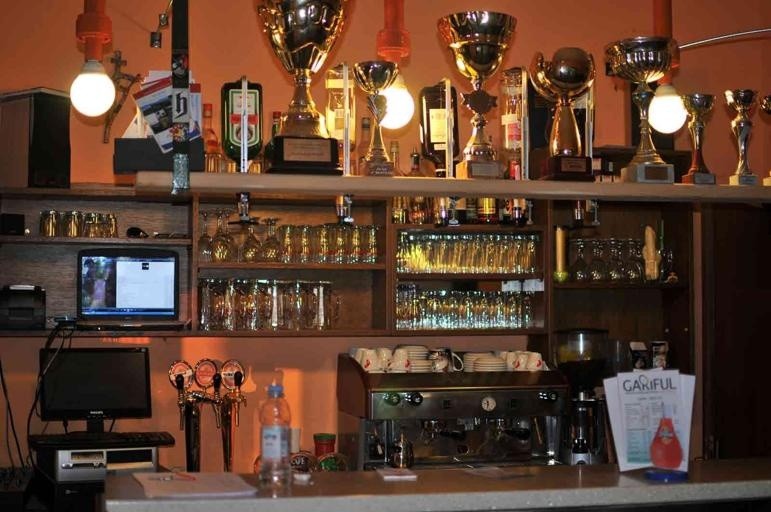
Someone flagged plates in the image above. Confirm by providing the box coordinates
[465,349,506,371]
[403,344,431,373]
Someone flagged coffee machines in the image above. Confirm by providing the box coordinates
[554,326,609,466]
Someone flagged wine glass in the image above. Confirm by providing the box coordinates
[569,237,678,282]
[201,206,282,262]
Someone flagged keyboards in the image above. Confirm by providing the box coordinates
[30,432,175,447]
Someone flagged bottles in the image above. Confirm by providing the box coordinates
[41,210,116,240]
[388,139,405,177]
[420,85,462,177]
[281,223,378,263]
[326,61,372,174]
[396,230,540,273]
[190,75,282,173]
[259,384,295,493]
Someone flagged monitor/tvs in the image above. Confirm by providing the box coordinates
[38,346,152,435]
[76,247,185,332]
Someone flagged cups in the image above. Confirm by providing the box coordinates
[428,349,467,371]
[350,345,410,374]
[496,349,545,371]
[200,276,343,331]
[395,283,533,329]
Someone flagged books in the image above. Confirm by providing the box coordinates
[376,466,416,481]
[601,366,696,474]
[132,471,259,498]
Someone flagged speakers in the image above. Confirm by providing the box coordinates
[0,87,71,189]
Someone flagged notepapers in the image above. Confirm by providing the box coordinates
[376,468,419,483]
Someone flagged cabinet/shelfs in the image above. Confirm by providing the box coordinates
[0,183,709,466]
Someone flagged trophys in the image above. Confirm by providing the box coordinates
[255,2,345,176]
[437,11,517,179]
[757,95,771,186]
[725,88,759,185]
[680,92,717,185]
[603,36,680,186]
[352,60,399,177]
[418,77,459,178]
[322,62,359,173]
[529,46,599,184]
